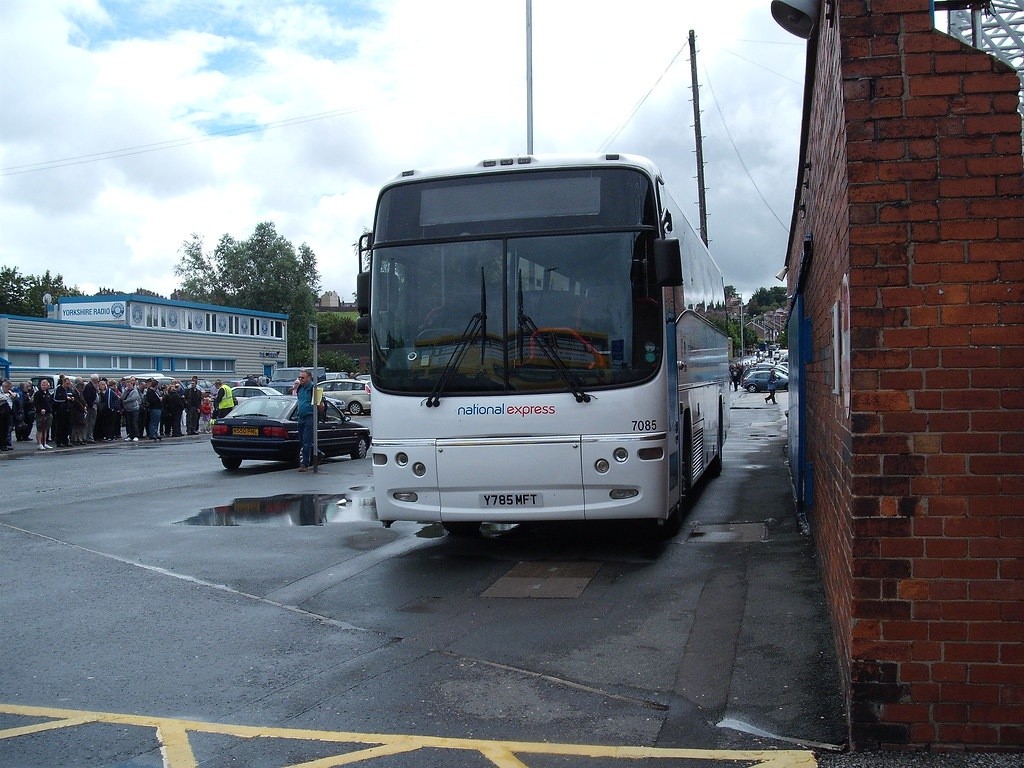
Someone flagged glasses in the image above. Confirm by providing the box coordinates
[299,377,308,379]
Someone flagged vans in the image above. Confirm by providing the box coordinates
[31,373,78,401]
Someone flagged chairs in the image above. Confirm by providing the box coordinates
[459,286,613,324]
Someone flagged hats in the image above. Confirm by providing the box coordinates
[212,379,222,386]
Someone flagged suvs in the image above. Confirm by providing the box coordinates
[122,373,212,398]
[739,361,789,393]
[316,378,371,416]
[266,366,326,394]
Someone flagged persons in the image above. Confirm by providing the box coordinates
[728,362,745,391]
[297,371,326,472]
[764,370,778,404]
[0,372,356,452]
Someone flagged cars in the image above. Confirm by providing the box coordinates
[210,395,371,470]
[326,372,371,390]
[231,385,284,405]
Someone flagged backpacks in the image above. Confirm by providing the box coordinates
[296,384,328,424]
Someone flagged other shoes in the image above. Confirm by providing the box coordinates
[73,441,87,446]
[44,444,53,448]
[23,437,33,441]
[298,464,306,472]
[37,444,46,449]
[0,446,13,451]
[17,438,23,442]
[188,431,199,435]
[57,443,73,447]
[150,435,164,439]
[103,438,118,442]
[204,431,211,433]
[124,437,132,441]
[133,437,139,441]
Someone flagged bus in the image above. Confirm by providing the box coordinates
[356,153,730,539]
